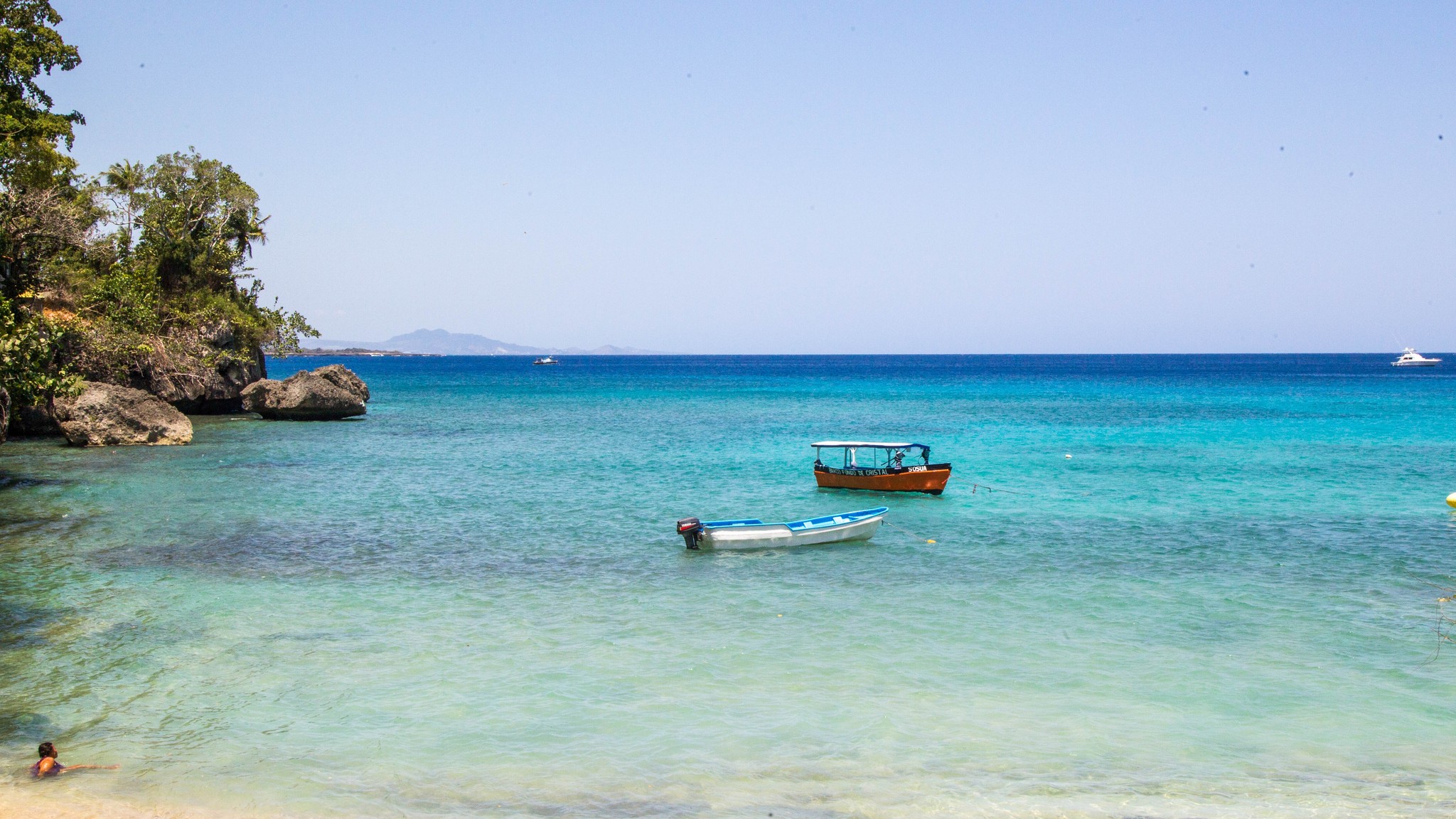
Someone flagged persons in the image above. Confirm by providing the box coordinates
[33,743,120,776]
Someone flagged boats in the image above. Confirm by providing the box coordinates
[532,354,558,366]
[1390,349,1443,367]
[674,503,891,556]
[808,440,953,494]
